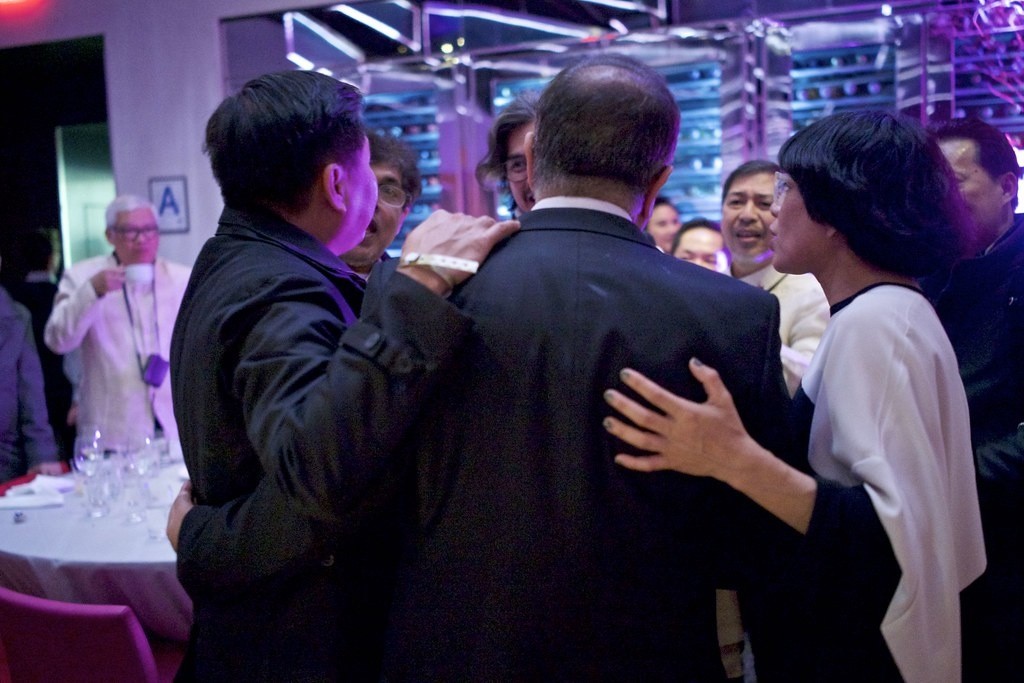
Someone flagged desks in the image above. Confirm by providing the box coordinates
[0,446,198,642]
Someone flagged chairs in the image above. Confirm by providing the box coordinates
[0,585,185,683]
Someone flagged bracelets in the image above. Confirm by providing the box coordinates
[397,252,480,299]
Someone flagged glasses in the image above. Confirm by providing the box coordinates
[772,171,803,205]
[378,184,412,208]
[112,225,159,242]
[502,155,527,182]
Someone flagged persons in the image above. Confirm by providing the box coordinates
[0,70,1024,682]
[165,49,790,683]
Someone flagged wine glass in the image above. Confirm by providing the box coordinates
[73,423,191,540]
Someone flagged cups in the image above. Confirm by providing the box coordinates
[119,263,153,286]
